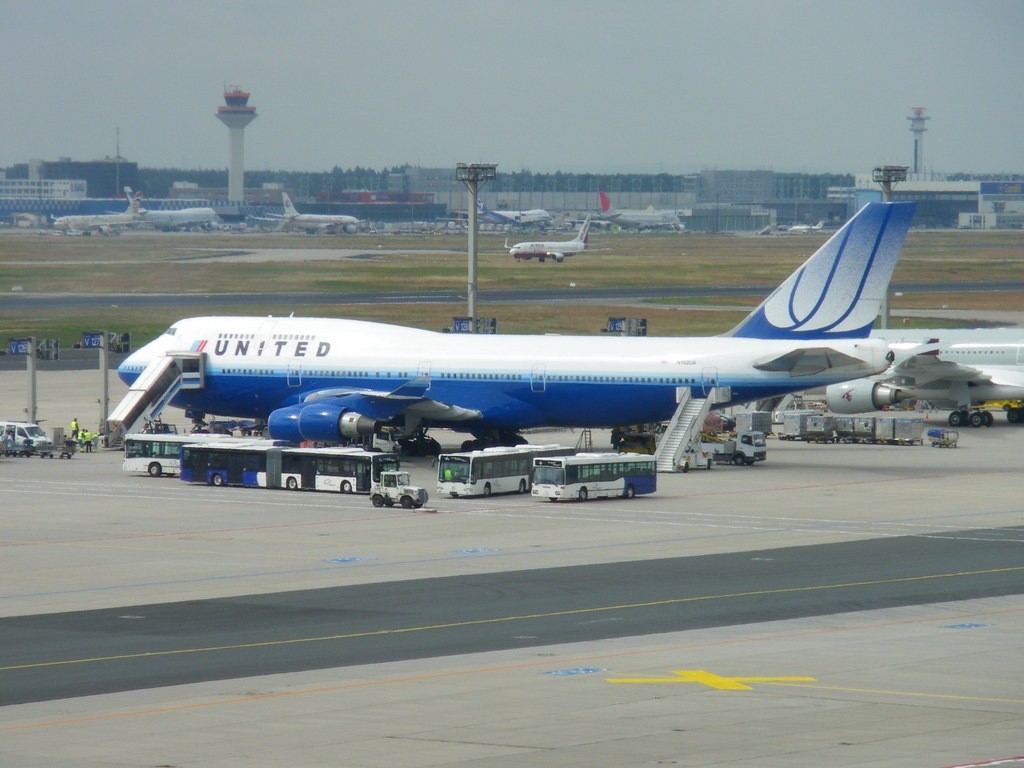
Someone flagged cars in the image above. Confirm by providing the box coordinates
[369,469,428,509]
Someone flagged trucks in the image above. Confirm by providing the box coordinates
[927,429,959,448]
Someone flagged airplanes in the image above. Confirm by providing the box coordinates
[771,327,1024,427]
[504,213,594,264]
[281,191,361,235]
[123,184,222,232]
[598,191,687,232]
[475,198,557,232]
[788,220,825,234]
[52,190,142,237]
[110,198,920,472]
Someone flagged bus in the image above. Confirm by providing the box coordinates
[431,444,575,500]
[122,433,291,477]
[180,442,401,495]
[531,452,657,501]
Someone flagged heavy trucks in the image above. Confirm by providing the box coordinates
[657,429,767,465]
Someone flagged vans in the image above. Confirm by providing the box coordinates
[0,421,47,448]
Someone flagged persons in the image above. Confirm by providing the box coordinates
[77,428,86,453]
[92,432,99,453]
[6,432,15,448]
[70,417,79,440]
[84,429,92,453]
[443,467,452,481]
